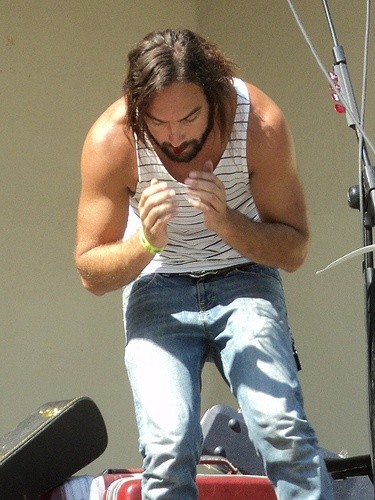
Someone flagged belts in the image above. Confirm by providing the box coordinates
[162,264,246,277]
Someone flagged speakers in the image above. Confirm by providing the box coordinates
[198,404,341,477]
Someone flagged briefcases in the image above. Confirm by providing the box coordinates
[0,396,375,499]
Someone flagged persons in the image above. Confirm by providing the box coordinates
[73,29,338,500]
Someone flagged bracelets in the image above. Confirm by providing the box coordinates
[138,227,167,254]
